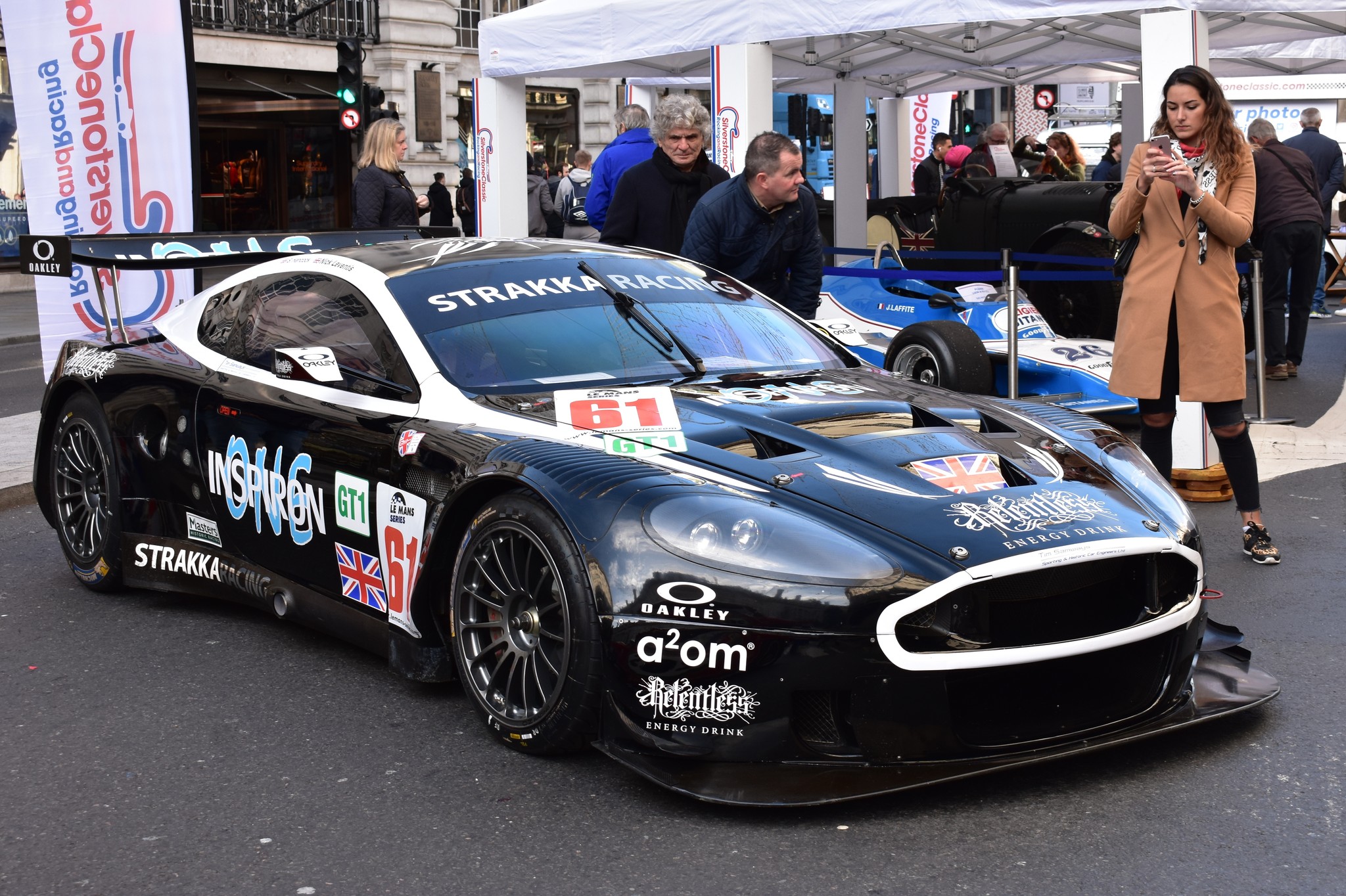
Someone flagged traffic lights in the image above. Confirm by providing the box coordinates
[367,84,401,130]
[334,37,363,133]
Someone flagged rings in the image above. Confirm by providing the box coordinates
[1153,165,1156,172]
[1184,161,1188,168]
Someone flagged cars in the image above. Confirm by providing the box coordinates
[800,163,1265,358]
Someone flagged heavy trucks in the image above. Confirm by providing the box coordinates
[772,91,878,198]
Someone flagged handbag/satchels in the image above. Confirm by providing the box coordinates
[1114,217,1142,277]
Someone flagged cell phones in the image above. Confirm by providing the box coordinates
[1031,143,1048,152]
[1150,135,1172,175]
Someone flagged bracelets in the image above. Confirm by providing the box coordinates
[1190,187,1205,205]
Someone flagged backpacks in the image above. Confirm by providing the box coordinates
[456,182,476,216]
[561,175,592,226]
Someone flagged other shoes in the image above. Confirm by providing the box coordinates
[1286,360,1297,377]
[1254,363,1288,380]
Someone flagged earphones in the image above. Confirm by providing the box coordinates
[939,147,941,151]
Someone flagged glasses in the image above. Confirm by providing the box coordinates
[1053,132,1070,146]
[988,136,1007,144]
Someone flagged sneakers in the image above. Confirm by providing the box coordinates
[1242,521,1280,564]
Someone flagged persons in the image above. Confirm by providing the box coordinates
[598,91,730,256]
[351,116,434,230]
[680,132,823,320]
[553,149,602,242]
[526,150,570,239]
[1012,130,1086,182]
[1247,117,1324,381]
[914,132,953,197]
[942,103,1022,210]
[584,102,659,235]
[1107,64,1282,565]
[1091,131,1121,182]
[1282,107,1346,318]
[455,167,476,237]
[427,172,455,227]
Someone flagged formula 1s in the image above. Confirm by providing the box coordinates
[810,240,1173,418]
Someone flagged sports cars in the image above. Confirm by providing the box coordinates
[20,224,1282,809]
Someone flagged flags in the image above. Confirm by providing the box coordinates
[0,0,197,385]
